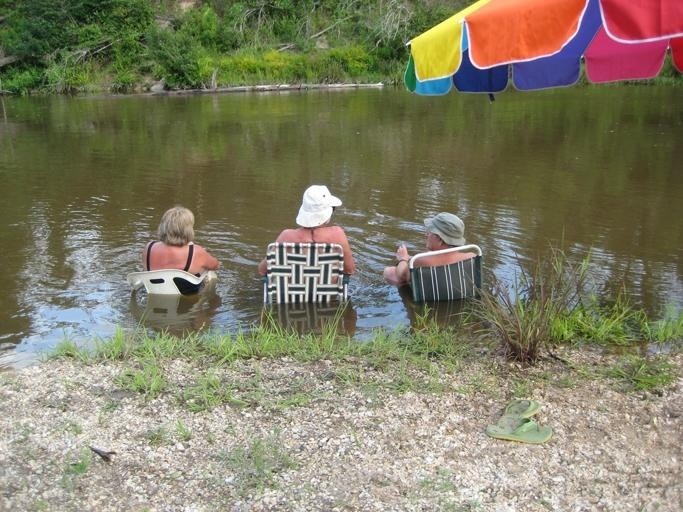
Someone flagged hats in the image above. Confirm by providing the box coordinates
[424,211,466,246]
[296,185,342,228]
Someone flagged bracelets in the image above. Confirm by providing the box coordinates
[398,260,407,263]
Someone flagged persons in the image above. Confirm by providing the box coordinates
[382,211,476,288]
[257,183,356,286]
[141,204,219,296]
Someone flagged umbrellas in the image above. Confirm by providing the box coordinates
[402,1,683,104]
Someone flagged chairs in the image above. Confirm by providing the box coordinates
[262,242,349,302]
[127,268,217,296]
[408,244,484,302]
